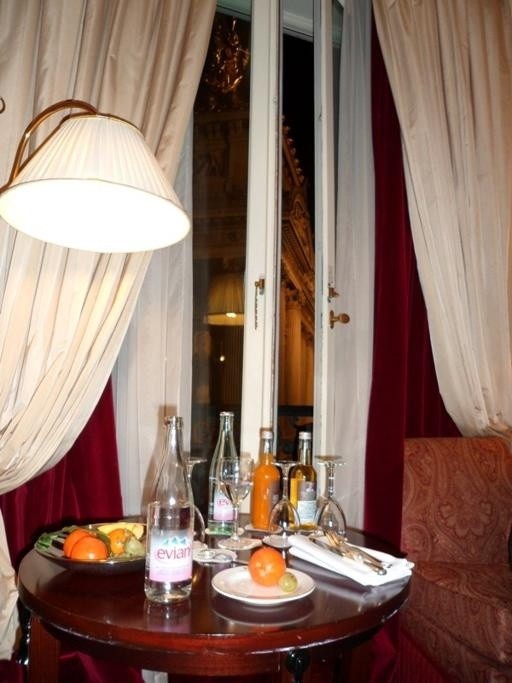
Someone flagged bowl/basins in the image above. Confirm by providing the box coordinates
[35,520,145,576]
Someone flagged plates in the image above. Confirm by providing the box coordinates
[210,565,315,605]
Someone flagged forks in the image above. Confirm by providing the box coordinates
[326,531,383,564]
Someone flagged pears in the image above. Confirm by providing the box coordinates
[123,525,146,558]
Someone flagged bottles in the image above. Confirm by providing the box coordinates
[206,412,319,537]
[147,414,195,604]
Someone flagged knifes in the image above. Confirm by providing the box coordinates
[306,538,385,578]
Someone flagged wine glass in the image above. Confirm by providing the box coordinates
[318,458,348,539]
[182,456,210,547]
[267,459,301,545]
[220,457,255,550]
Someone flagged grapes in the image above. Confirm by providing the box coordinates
[279,574,297,592]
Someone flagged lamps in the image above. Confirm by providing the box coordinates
[0,97,192,255]
[202,272,244,326]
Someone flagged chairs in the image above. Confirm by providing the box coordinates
[399,434,511,683]
